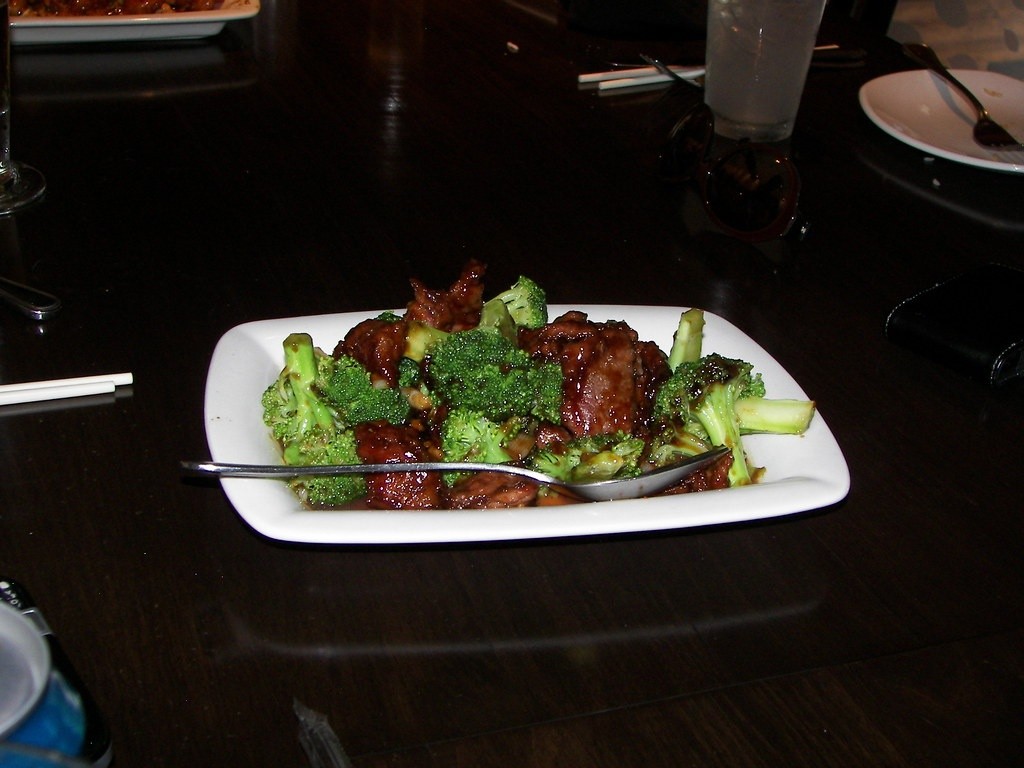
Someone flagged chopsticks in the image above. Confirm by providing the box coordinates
[578,65,707,90]
[0,372,133,406]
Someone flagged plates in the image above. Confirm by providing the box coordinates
[12,0,261,47]
[204,303,850,543]
[858,68,1024,173]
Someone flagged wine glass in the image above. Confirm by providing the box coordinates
[0,0,47,217]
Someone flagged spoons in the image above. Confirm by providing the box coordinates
[180,445,735,499]
[640,49,865,89]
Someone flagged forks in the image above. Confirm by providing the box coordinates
[903,39,1024,149]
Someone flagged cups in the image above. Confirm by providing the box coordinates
[704,0,826,143]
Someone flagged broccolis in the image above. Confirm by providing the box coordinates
[260,274,816,505]
